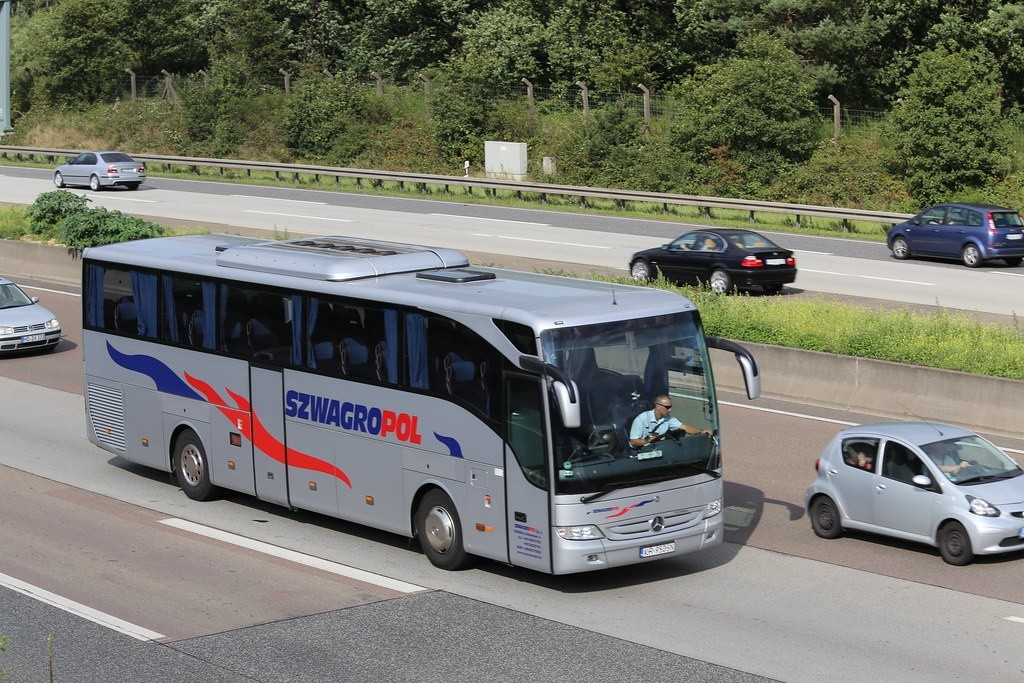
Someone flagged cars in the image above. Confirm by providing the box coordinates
[52,150,147,191]
[886,202,1024,268]
[629,228,797,296]
[805,420,1024,567]
[0,278,63,356]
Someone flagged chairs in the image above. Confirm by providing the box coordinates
[104,295,489,399]
[705,239,717,249]
[606,374,653,453]
[886,444,915,482]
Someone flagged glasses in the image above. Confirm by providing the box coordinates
[657,403,672,410]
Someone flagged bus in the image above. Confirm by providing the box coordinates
[81,233,762,577]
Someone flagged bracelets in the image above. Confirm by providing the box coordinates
[700,429,703,434]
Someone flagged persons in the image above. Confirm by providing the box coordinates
[680,235,708,250]
[921,441,971,489]
[854,451,871,469]
[628,395,713,449]
